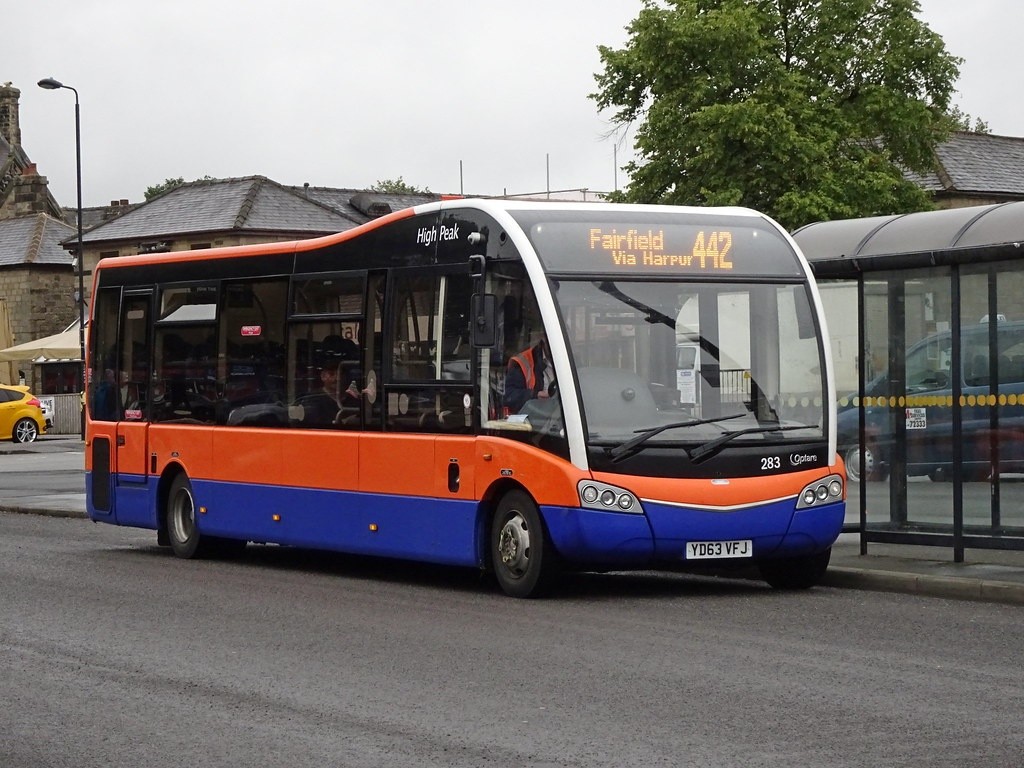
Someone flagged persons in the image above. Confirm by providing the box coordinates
[503,319,570,415]
[96,333,358,430]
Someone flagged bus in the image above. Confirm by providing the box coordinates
[85,196,848,600]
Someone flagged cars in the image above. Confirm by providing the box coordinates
[0,382,48,444]
[836,313,1023,485]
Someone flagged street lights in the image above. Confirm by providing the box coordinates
[36,76,85,442]
